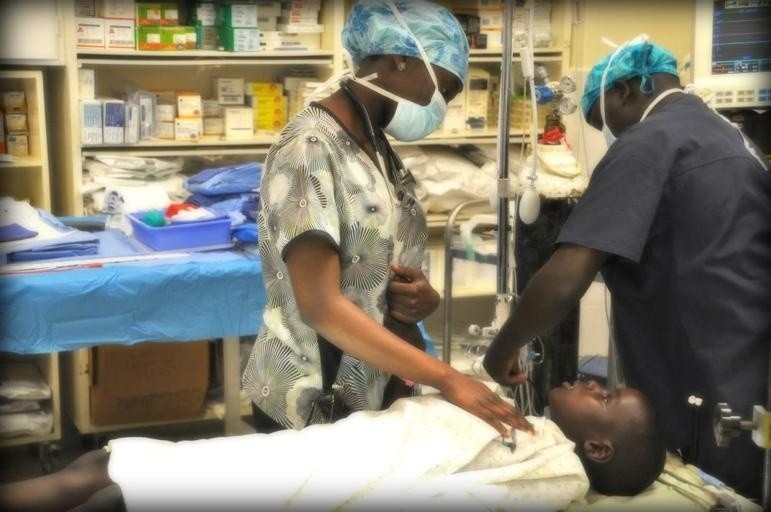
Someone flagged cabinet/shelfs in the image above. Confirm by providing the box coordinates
[74,1,344,434]
[72,60,341,147]
[349,3,568,304]
[67,0,343,60]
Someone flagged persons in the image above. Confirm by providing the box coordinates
[239,1,537,443]
[4,372,670,510]
[482,36,771,507]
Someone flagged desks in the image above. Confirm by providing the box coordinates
[3,249,274,357]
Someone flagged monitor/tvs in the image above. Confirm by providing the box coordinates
[693,0,771,110]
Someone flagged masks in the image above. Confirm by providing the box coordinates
[355,27,448,143]
[599,86,653,146]
